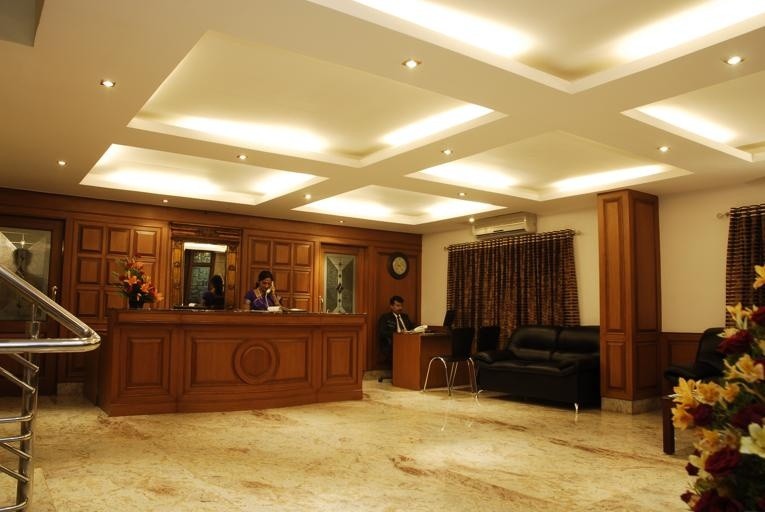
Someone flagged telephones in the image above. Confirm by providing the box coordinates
[414,325,428,333]
[266,281,274,294]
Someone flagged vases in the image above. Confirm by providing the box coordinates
[127,293,144,308]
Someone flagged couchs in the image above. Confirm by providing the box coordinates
[478,325,599,412]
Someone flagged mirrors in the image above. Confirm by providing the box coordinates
[164,222,243,310]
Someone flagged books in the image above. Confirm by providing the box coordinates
[403,323,427,334]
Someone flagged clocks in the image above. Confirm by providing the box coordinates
[388,253,410,279]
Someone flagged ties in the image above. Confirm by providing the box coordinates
[396,316,404,332]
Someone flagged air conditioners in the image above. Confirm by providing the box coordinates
[472,212,537,241]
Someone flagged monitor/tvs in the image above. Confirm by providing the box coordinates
[443,309,457,326]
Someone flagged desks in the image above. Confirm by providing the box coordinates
[392,331,476,390]
[660,395,682,455]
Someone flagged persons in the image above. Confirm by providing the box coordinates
[201,273,225,311]
[1,246,44,317]
[243,268,283,312]
[375,294,415,371]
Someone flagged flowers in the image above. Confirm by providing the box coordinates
[115,255,164,303]
[669,265,765,512]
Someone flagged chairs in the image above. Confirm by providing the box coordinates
[661,328,765,393]
[452,325,499,393]
[423,327,475,396]
[377,336,393,382]
